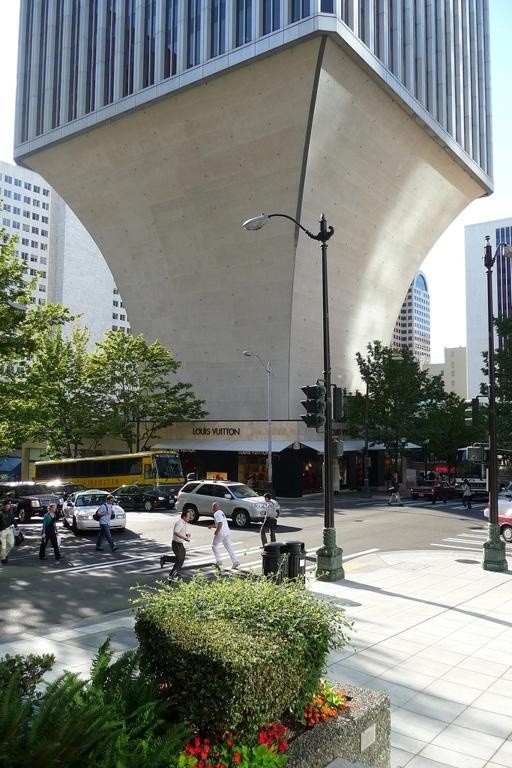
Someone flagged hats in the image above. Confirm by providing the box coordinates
[3,500,12,505]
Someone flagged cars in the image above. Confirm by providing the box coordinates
[497,507,512,542]
[61,488,126,533]
[109,481,175,510]
[410,479,454,500]
[484,498,512,521]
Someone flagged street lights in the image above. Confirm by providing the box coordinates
[480,234,511,526]
[243,206,350,583]
[241,348,276,491]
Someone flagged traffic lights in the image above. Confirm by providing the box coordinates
[462,398,475,428]
[299,381,327,430]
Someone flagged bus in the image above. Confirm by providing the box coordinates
[452,443,512,496]
[32,449,187,494]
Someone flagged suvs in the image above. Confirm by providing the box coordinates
[37,483,88,505]
[0,481,64,522]
[173,478,282,526]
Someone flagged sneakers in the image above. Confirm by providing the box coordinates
[160,554,166,569]
[232,560,240,570]
[95,545,120,552]
[39,556,64,560]
[215,560,224,567]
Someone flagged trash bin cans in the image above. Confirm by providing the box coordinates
[261,541,291,588]
[286,540,308,584]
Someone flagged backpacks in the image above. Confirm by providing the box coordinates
[92,502,109,521]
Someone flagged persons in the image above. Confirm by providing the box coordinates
[161,512,190,578]
[461,479,471,510]
[260,494,277,549]
[0,503,18,563]
[247,476,256,488]
[40,504,64,560]
[96,495,119,551]
[208,502,240,569]
[418,471,436,485]
[388,473,404,506]
